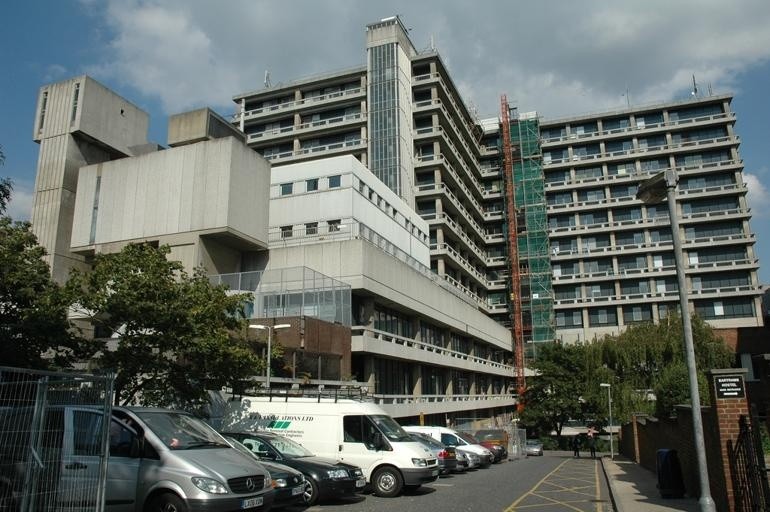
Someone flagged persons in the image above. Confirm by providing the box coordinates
[589,440,598,459]
[573,437,582,459]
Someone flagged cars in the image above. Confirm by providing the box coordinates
[401,425,510,476]
[220,431,367,512]
[522,439,544,457]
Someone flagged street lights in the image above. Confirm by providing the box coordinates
[600,383,614,461]
[634,169,717,512]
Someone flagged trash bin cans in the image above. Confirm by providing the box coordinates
[656,449,687,500]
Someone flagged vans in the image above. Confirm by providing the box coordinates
[202,393,440,498]
[0,405,275,512]
